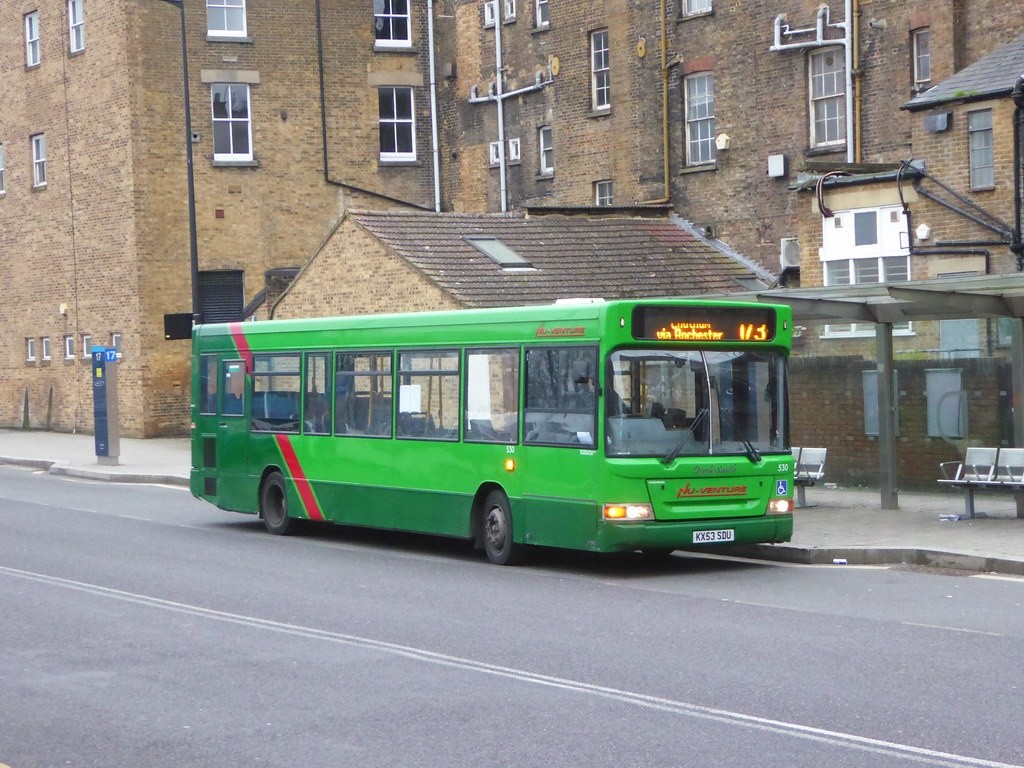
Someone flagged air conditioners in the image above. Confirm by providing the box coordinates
[781,237,800,271]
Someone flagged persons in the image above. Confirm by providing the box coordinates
[575,372,629,419]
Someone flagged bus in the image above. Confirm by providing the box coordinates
[186,300,795,565]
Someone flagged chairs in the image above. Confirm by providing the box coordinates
[794,447,827,480]
[1002,464,1024,486]
[264,390,291,419]
[937,447,998,483]
[352,391,380,429]
[791,447,801,468]
[240,392,264,419]
[377,391,392,422]
[208,392,238,414]
[968,447,1024,485]
[409,411,436,437]
[470,419,496,440]
